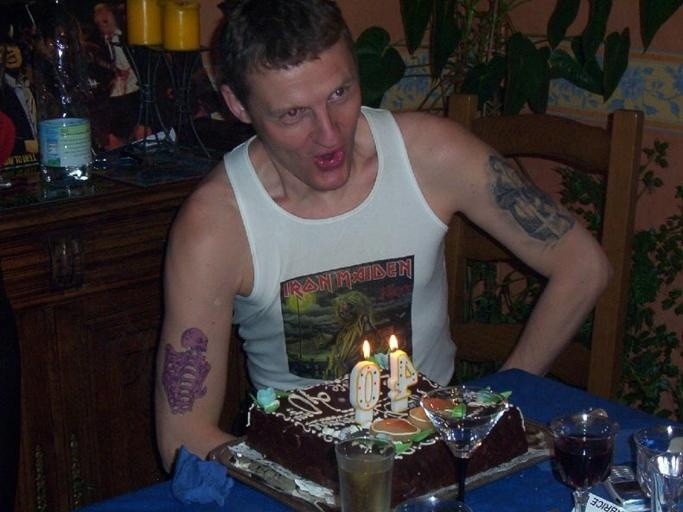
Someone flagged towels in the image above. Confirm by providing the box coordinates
[172,447,234,507]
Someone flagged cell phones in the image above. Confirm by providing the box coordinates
[603,464,646,508]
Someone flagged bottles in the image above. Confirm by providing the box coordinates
[31,1,94,188]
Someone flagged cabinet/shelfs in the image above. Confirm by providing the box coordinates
[1,159,257,511]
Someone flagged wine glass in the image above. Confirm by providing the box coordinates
[420,387,508,510]
[550,413,621,511]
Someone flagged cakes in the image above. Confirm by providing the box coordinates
[244,363,529,512]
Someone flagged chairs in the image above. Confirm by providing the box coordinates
[444,94,646,399]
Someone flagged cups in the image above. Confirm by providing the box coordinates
[648,452,682,512]
[632,425,683,499]
[335,438,395,512]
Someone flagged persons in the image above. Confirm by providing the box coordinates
[88,2,157,152]
[0,35,49,152]
[153,1,619,479]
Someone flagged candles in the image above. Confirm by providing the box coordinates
[125,0,201,51]
[386,334,419,413]
[349,340,381,428]
